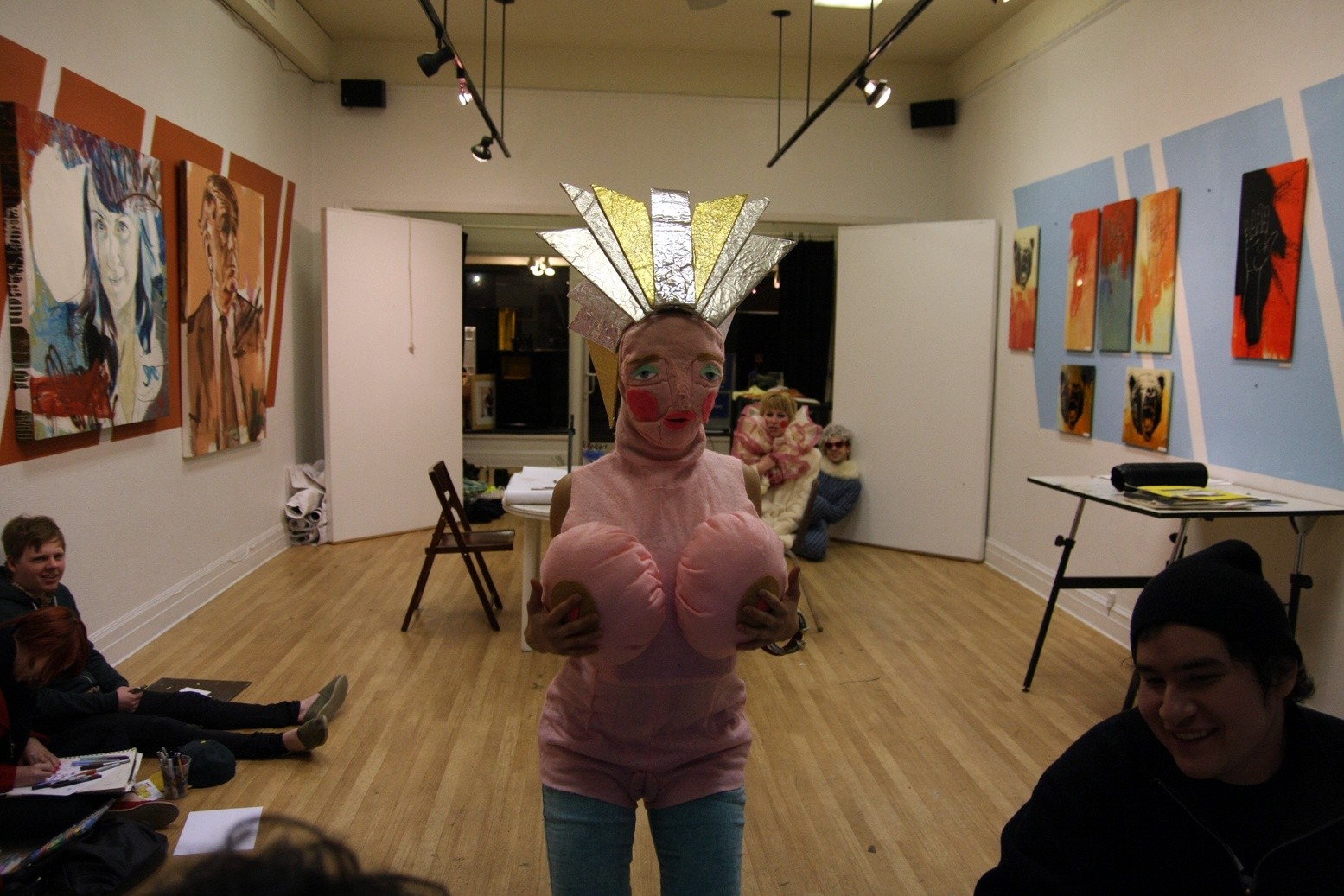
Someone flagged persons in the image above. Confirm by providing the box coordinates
[523,308,862,896]
[975,539,1344,895]
[0,517,447,896]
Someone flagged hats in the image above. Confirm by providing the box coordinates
[1130,538,1302,664]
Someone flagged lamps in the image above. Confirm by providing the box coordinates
[767,0,933,167]
[528,254,555,276]
[416,0,514,163]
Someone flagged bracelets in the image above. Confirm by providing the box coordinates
[764,611,807,656]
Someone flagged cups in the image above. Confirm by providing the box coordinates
[160,755,191,799]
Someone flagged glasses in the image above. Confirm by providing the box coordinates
[824,441,844,448]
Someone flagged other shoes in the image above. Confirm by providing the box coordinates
[304,674,349,722]
[292,715,328,758]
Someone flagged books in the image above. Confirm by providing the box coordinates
[6,747,143,798]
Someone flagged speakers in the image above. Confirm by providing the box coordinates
[340,78,387,108]
[909,98,957,129]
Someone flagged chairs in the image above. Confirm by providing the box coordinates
[399,461,516,632]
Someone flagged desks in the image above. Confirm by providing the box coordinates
[1020,476,1344,693]
[502,466,590,652]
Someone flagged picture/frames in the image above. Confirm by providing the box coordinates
[471,376,495,431]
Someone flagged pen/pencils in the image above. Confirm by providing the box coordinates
[130,684,148,692]
[31,763,121,789]
[156,746,187,798]
[30,753,38,763]
[71,755,130,770]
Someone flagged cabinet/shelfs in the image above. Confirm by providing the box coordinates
[496,349,568,435]
